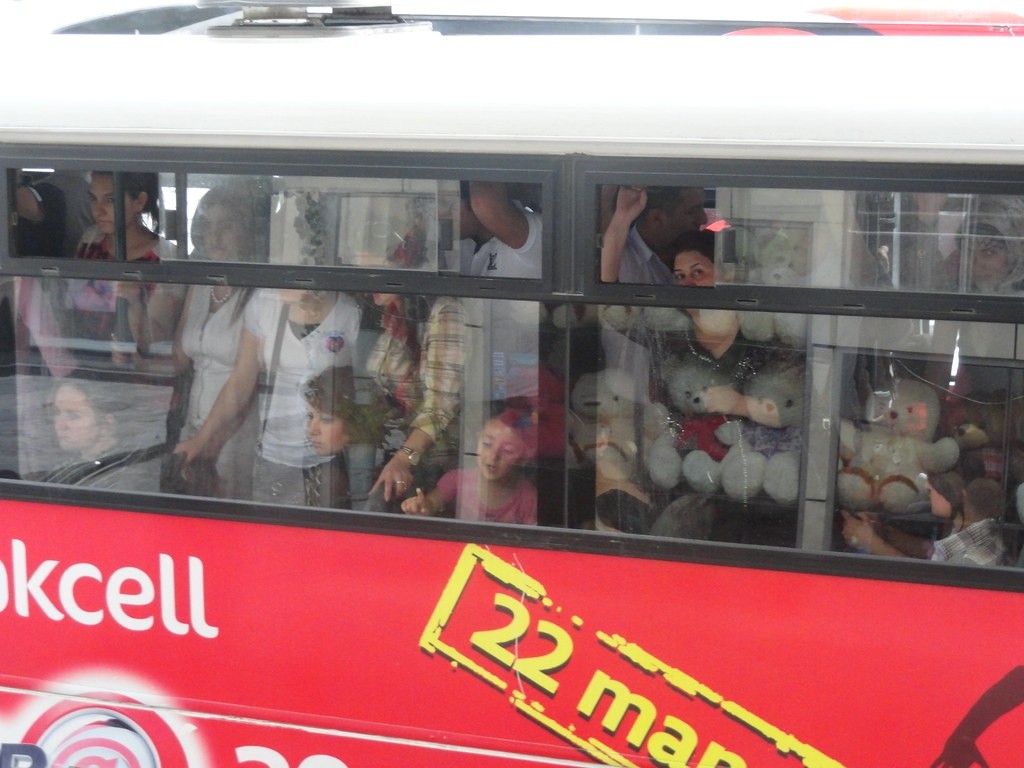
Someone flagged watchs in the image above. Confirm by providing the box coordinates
[400,446,419,465]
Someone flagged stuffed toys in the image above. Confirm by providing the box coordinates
[648,363,737,489]
[719,360,799,501]
[569,372,659,480]
[836,378,960,513]
[941,390,1024,517]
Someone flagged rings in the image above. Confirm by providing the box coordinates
[397,481,404,484]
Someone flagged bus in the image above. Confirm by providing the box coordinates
[0,0,1024,768]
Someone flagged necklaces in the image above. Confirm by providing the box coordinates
[211,286,231,303]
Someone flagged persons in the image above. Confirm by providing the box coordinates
[600,182,800,382]
[13,166,538,525]
[956,193,1024,296]
[840,477,1002,566]
